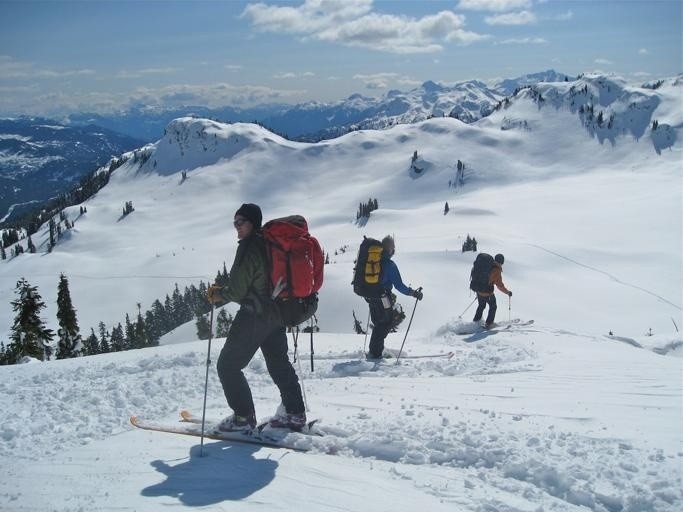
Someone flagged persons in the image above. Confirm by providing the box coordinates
[472,254,511,328]
[205,203,305,432]
[365,236,421,357]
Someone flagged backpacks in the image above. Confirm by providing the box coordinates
[256,215,324,324]
[352,236,385,297]
[470,254,494,292]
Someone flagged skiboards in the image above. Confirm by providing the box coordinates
[347,352,454,359]
[130,411,330,450]
[454,319,534,335]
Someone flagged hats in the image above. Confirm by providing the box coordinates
[382,238,394,250]
[235,203,262,229]
[495,254,504,263]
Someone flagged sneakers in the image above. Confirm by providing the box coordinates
[219,413,257,431]
[366,350,382,360]
[269,412,306,429]
[486,320,494,324]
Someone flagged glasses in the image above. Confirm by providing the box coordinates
[234,219,248,227]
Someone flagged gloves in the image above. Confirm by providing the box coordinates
[407,287,423,300]
[207,286,226,303]
[509,292,512,296]
